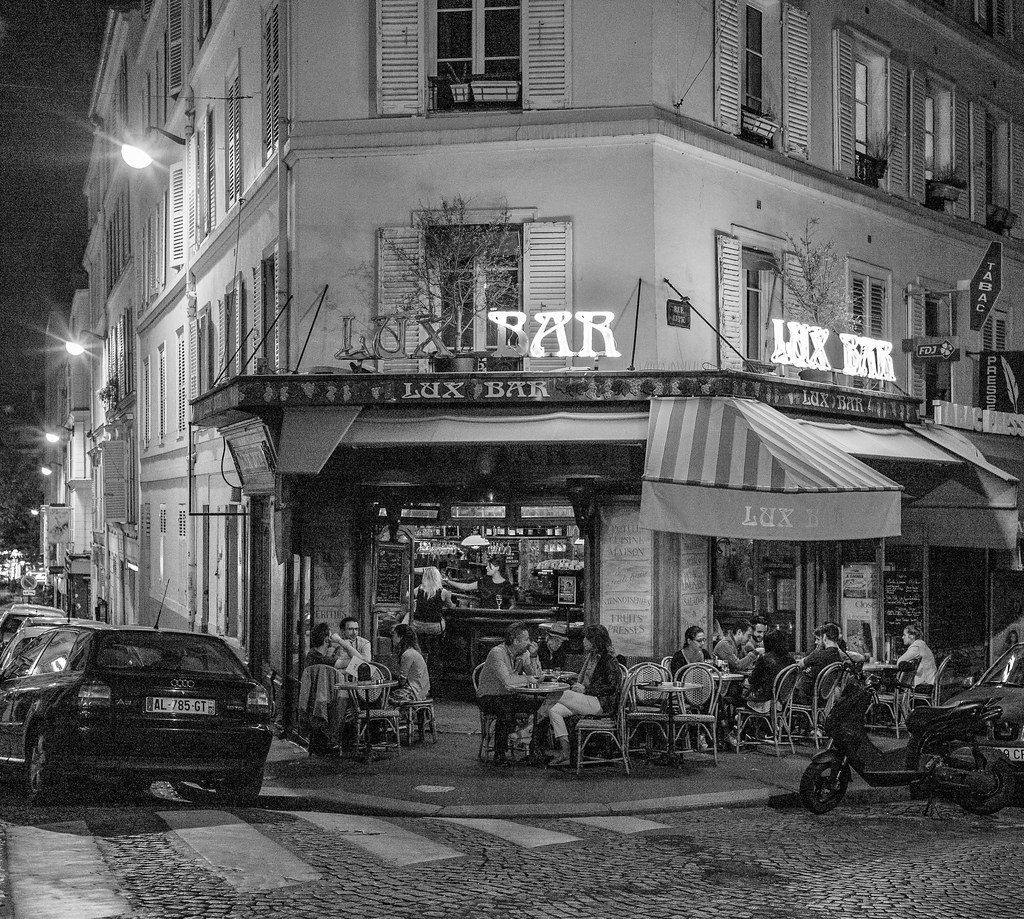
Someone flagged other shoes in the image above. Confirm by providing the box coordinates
[697,734,708,748]
[492,751,512,767]
[548,753,570,765]
[724,727,744,747]
[507,728,533,744]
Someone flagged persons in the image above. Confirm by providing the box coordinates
[388,625,430,700]
[448,558,515,610]
[1002,631,1018,652]
[897,621,937,693]
[413,566,456,634]
[476,622,620,765]
[797,622,865,707]
[672,616,797,714]
[305,618,371,669]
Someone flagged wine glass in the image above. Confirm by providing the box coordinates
[721,660,729,675]
[552,667,561,688]
[416,540,457,556]
[496,594,503,610]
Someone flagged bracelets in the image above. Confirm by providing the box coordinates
[530,655,537,658]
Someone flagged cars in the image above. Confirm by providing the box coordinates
[0,578,275,807]
[1,603,104,668]
[944,643,1024,797]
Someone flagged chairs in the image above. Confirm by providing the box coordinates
[295,653,960,776]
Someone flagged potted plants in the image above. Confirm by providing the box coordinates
[445,62,521,103]
[377,193,532,373]
[742,106,778,139]
[867,125,898,179]
[932,176,967,202]
[760,216,869,385]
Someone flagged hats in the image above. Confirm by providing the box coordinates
[546,624,569,640]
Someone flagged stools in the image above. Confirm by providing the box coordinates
[416,634,447,703]
[478,635,506,663]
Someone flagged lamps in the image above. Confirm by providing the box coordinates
[460,525,491,550]
[45,425,74,443]
[65,329,108,356]
[41,461,62,475]
[121,126,186,169]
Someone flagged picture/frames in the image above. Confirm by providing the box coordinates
[558,576,576,604]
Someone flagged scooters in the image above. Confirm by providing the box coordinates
[800,624,1015,816]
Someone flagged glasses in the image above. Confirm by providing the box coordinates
[692,637,707,642]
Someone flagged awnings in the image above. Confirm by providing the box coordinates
[793,419,1020,481]
[641,397,906,542]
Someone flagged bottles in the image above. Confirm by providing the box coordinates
[487,542,511,554]
